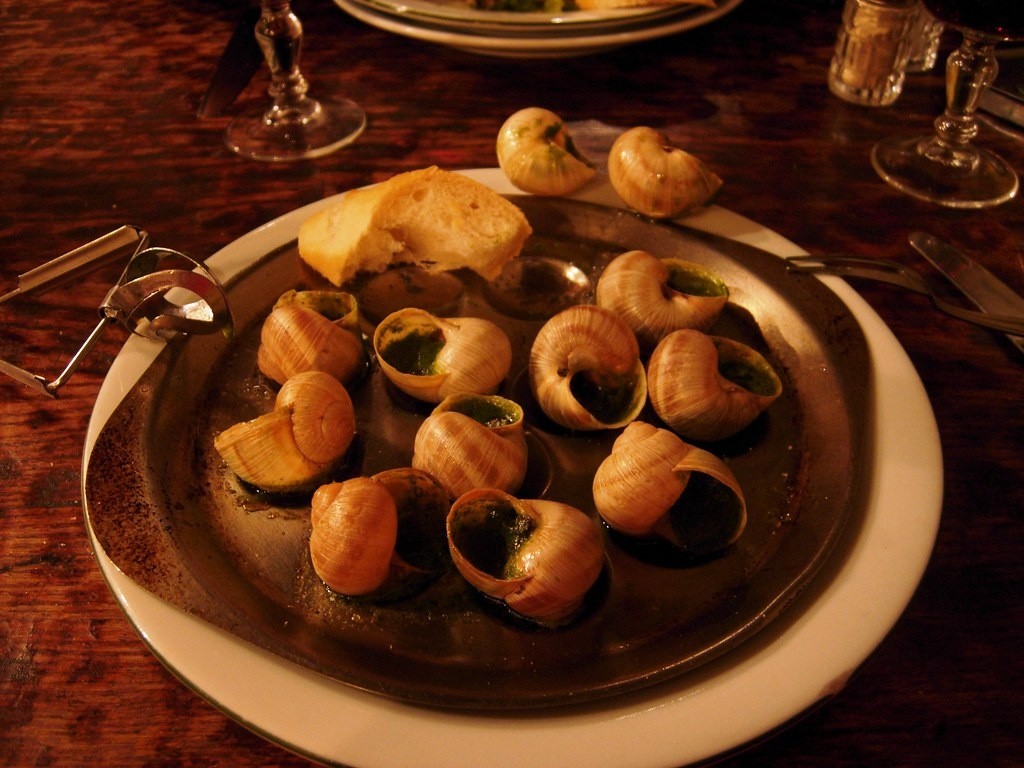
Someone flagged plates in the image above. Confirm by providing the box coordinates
[329,0,738,60]
[81,167,942,768]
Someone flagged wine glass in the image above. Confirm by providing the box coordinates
[221,0,367,160]
[869,0,1024,208]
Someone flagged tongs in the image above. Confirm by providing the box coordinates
[0,220,242,400]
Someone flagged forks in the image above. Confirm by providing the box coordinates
[782,255,1024,337]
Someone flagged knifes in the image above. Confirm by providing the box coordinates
[908,230,1024,355]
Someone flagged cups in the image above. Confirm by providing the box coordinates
[827,3,942,108]
